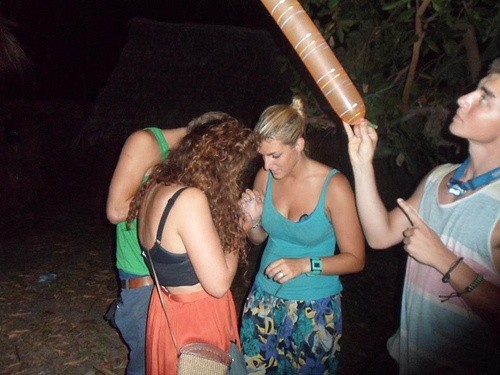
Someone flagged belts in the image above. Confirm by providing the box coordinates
[119,272,155,289]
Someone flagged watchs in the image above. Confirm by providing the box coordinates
[306,257,323,276]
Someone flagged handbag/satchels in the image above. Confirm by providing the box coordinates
[176,342,232,375]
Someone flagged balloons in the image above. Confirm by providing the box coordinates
[260,1,377,130]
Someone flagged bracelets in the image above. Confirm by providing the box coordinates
[252,216,262,228]
[441,257,464,283]
[438,272,484,303]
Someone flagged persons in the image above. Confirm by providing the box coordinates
[240,96,366,375]
[123,112,261,375]
[106,112,229,375]
[340,61,500,375]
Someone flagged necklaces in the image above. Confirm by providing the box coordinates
[446,155,499,196]
